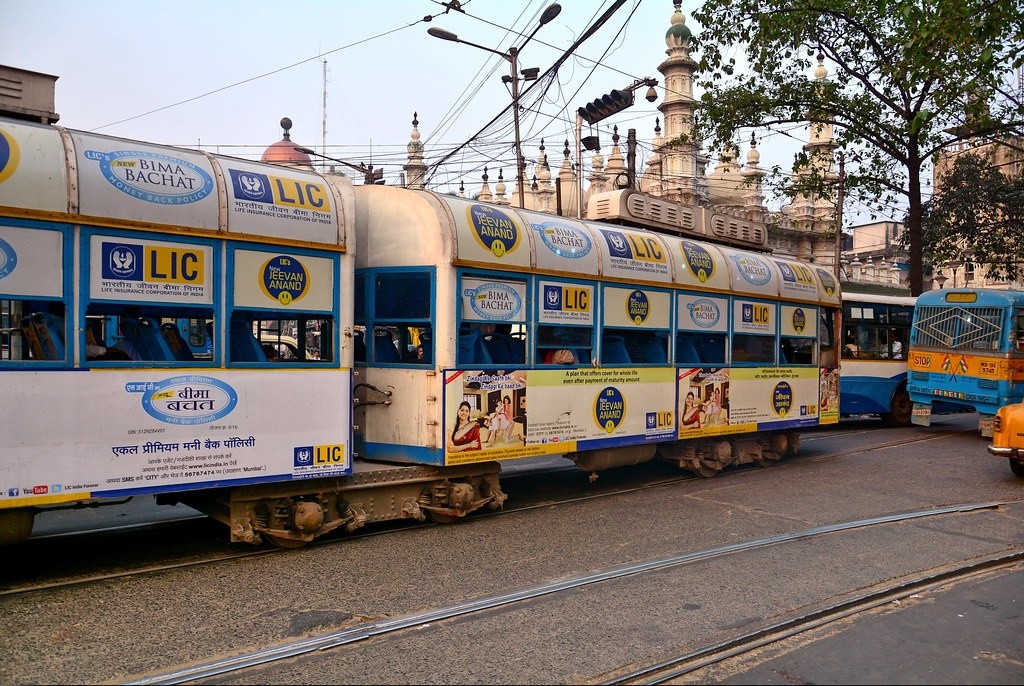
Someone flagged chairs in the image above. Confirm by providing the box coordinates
[353,327,794,365]
[8,311,274,361]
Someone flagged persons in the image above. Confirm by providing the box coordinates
[417,345,423,360]
[263,345,321,360]
[87,344,134,360]
[447,395,526,452]
[490,324,574,364]
[681,387,729,429]
[844,332,903,359]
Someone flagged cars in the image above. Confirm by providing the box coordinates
[254,335,319,360]
[987,394,1024,476]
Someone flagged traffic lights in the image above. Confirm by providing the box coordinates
[578,89,634,125]
[581,136,601,151]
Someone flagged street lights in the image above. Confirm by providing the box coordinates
[932,248,977,288]
[428,2,562,207]
[294,147,385,185]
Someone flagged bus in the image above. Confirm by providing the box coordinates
[0,119,842,550]
[906,288,1024,436]
[841,289,919,427]
